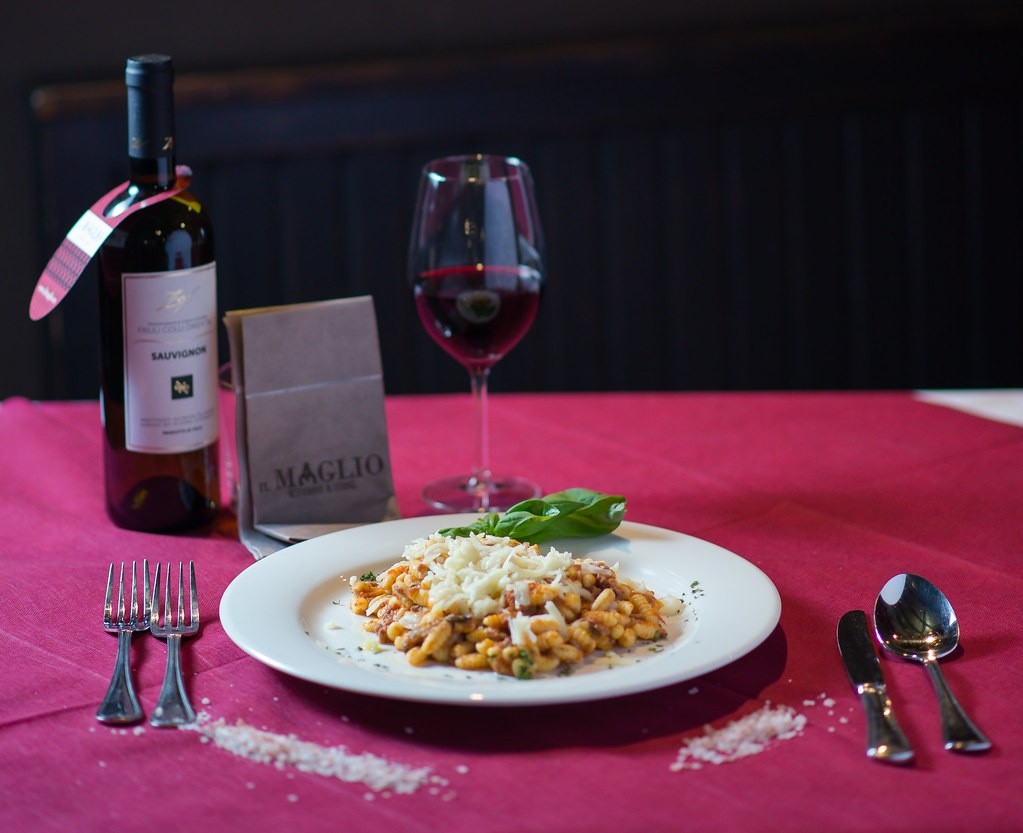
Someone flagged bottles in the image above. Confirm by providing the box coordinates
[95,53,224,533]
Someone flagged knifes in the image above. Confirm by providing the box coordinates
[837,609,916,760]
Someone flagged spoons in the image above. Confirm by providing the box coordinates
[873,571,994,749]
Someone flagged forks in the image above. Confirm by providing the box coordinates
[149,560,203,728]
[98,561,154,725]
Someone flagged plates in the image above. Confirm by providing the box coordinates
[219,512,783,708]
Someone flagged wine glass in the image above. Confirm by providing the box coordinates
[417,153,544,513]
